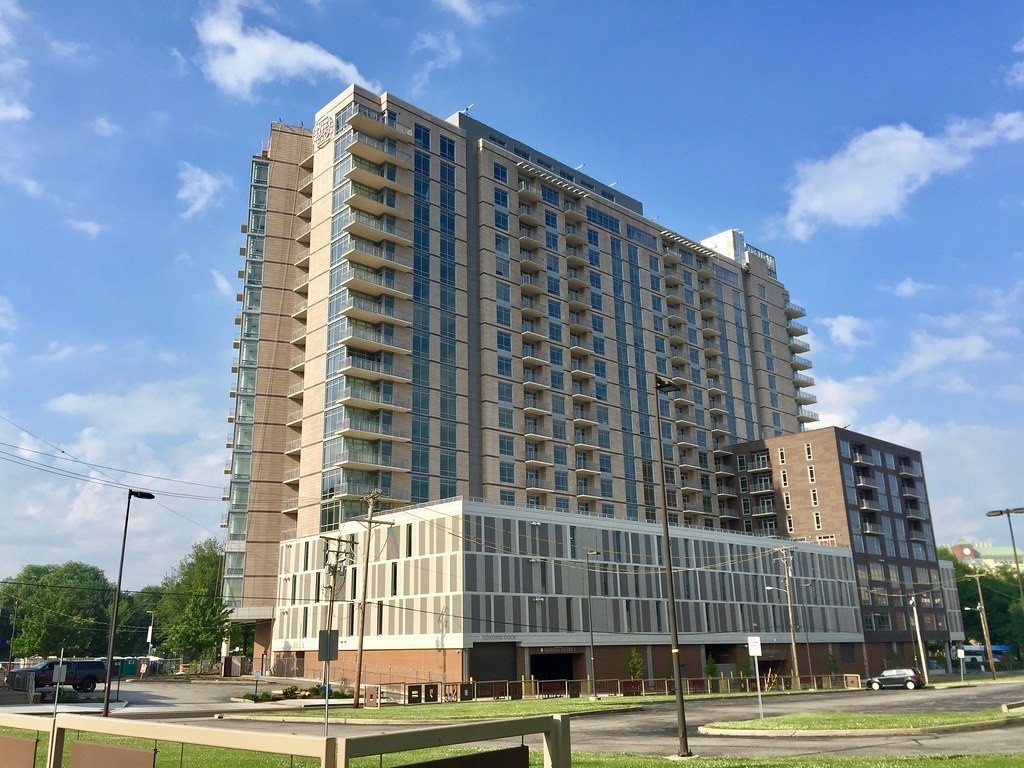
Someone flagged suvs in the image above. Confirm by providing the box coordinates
[866,667,925,691]
[9,659,107,693]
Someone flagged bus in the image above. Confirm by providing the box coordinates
[949,644,1011,668]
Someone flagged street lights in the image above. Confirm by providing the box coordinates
[986,508,1024,602]
[653,372,695,757]
[964,602,997,680]
[102,489,156,718]
[585,549,602,697]
[146,610,156,656]
[764,587,801,691]
[323,557,346,736]
[801,583,814,690]
